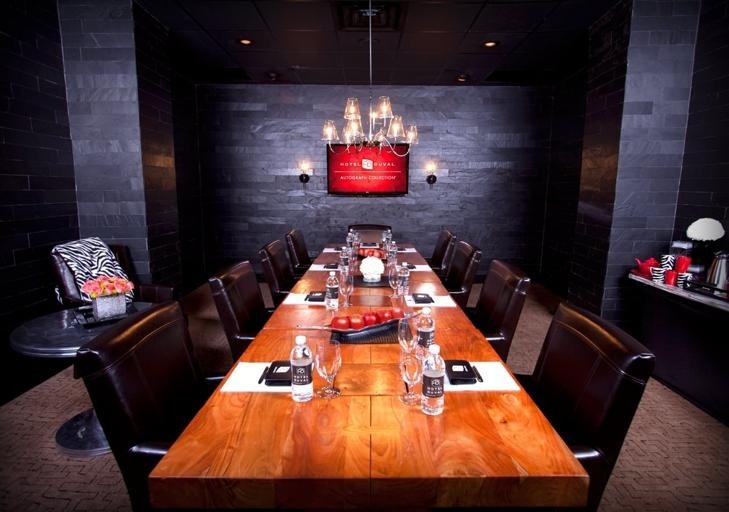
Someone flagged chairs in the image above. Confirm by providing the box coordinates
[512,302,656,511]
[105,244,174,307]
[75,300,226,511]
[348,224,392,244]
[209,228,317,360]
[426,231,529,363]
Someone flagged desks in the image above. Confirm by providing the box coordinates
[619,272,729,428]
[9,302,157,456]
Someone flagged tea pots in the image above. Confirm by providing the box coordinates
[632,256,661,280]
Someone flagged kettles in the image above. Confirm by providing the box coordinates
[704,249,729,299]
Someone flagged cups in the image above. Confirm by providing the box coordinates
[649,254,693,290]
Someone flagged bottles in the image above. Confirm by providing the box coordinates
[290,336,314,403]
[421,343,446,415]
[325,228,409,310]
[415,307,435,348]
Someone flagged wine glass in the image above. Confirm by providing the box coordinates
[399,356,422,406]
[398,316,418,369]
[315,339,342,399]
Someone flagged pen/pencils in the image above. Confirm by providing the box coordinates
[304,294,309,301]
[472,366,483,384]
[257,366,269,383]
[324,265,326,268]
[431,298,434,302]
[414,266,416,269]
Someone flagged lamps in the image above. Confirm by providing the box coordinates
[299,159,310,189]
[425,160,437,189]
[321,0,419,159]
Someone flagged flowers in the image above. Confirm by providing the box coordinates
[81,274,134,300]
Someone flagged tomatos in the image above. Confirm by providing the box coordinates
[358,247,385,258]
[331,308,403,330]
